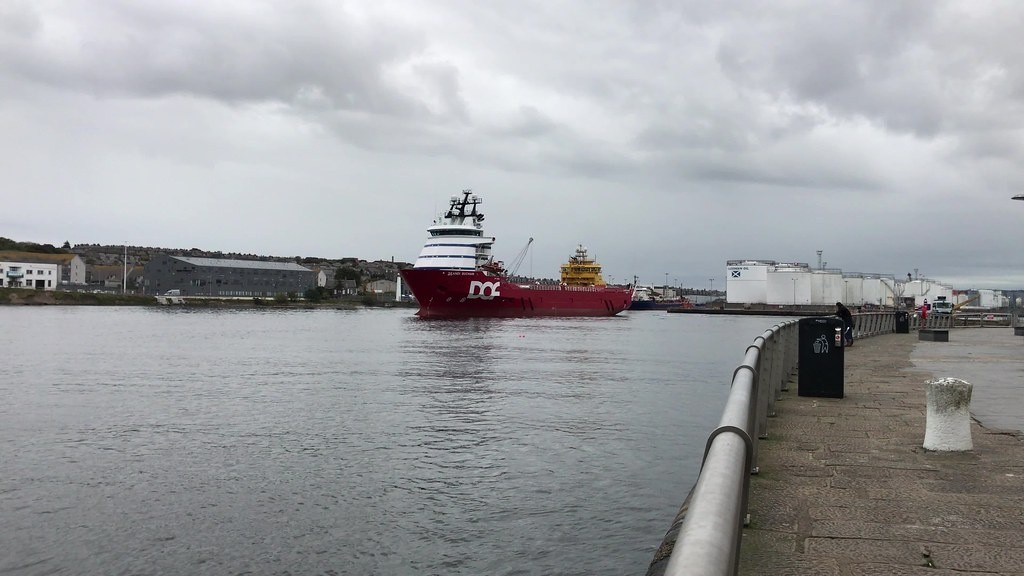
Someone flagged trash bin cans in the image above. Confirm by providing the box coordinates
[799,317,843,399]
[896,311,908,334]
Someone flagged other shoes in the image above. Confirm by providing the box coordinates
[844,344,851,347]
[848,339,853,345]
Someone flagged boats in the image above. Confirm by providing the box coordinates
[628,286,695,312]
[396,188,636,319]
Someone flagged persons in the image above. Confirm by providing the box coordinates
[835,302,853,347]
[919,304,928,330]
